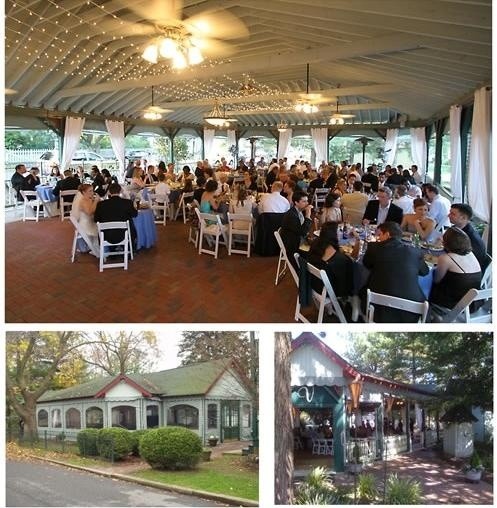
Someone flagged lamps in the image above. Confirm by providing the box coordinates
[139,25,205,71]
[291,102,320,114]
[203,96,227,126]
[329,116,344,125]
[143,110,162,121]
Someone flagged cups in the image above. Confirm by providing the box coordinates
[347,189,379,199]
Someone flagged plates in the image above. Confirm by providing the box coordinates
[41,180,53,187]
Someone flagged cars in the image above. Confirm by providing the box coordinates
[124,149,153,166]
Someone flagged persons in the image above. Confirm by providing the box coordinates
[317,417,415,439]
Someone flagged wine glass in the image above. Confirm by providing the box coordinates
[93,192,101,200]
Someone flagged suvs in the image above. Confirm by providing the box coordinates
[36,149,109,173]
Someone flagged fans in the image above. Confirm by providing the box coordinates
[326,99,357,125]
[287,61,339,113]
[139,84,174,122]
[96,0,249,73]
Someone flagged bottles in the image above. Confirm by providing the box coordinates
[313,221,444,259]
[26,170,37,174]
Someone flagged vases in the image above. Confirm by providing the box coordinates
[207,438,217,447]
[198,451,212,462]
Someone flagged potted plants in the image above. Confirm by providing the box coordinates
[458,445,485,480]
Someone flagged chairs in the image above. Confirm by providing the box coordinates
[295,429,373,461]
[10,162,491,322]
